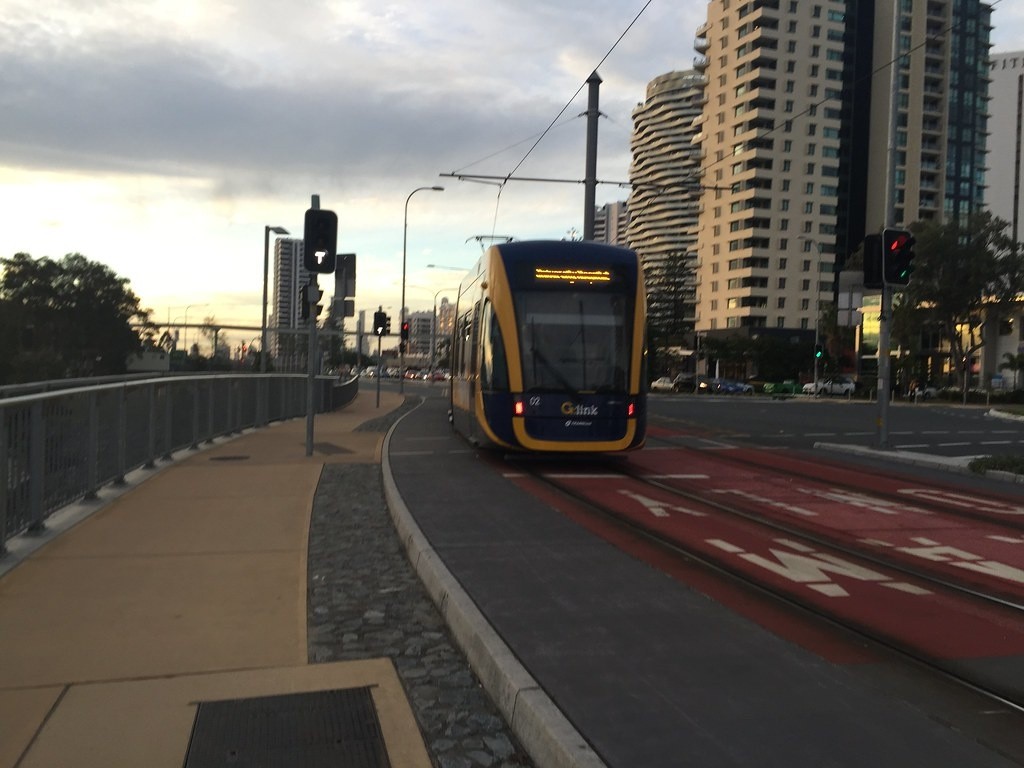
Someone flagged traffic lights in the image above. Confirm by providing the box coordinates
[374,312,386,336]
[243,343,246,352]
[303,209,337,274]
[885,229,917,284]
[814,344,823,359]
[401,322,408,341]
[386,317,391,335]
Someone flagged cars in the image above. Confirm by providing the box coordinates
[802,374,856,398]
[650,368,796,395]
[328,364,450,380]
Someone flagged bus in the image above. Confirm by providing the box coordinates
[447,234,651,457]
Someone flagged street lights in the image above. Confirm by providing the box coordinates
[397,185,446,395]
[796,235,821,396]
[262,225,290,374]
